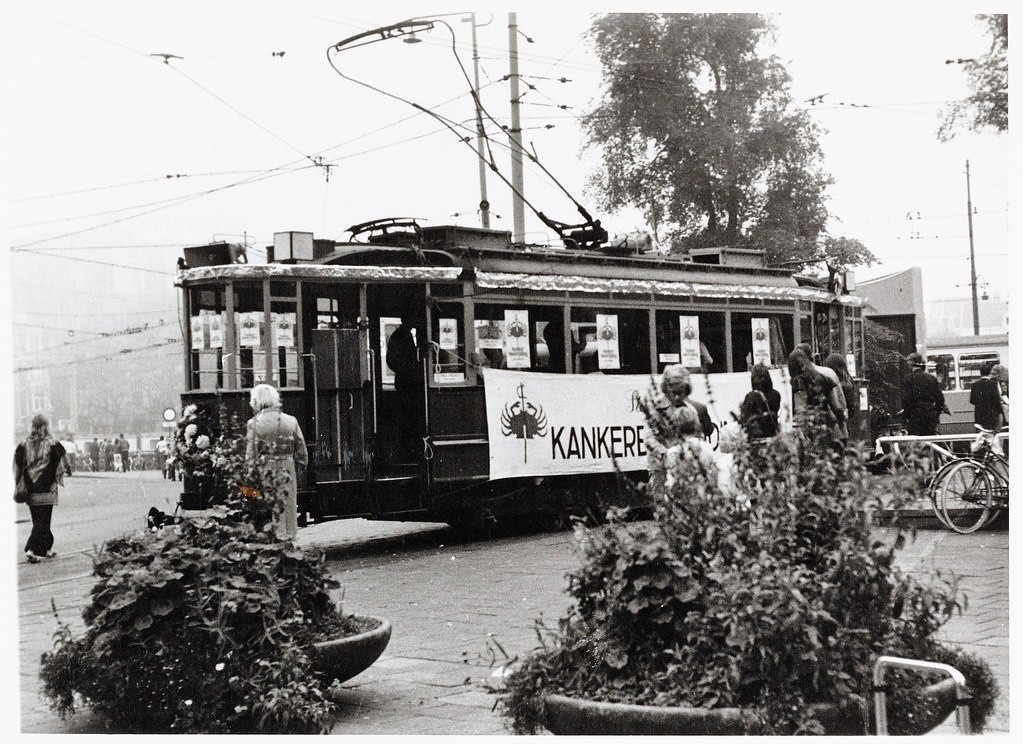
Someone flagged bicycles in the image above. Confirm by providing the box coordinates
[926,424,1009,535]
[75,449,155,473]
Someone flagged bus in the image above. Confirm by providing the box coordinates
[172,16,869,541]
[924,331,1010,458]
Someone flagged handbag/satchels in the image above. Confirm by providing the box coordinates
[13,440,28,503]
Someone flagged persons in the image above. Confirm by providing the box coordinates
[674,339,713,373]
[787,343,860,472]
[13,414,72,562]
[386,307,430,458]
[155,435,169,479]
[645,365,719,510]
[543,307,581,373]
[970,363,1009,475]
[245,384,308,544]
[902,353,945,485]
[59,436,76,471]
[90,433,130,472]
[739,366,782,488]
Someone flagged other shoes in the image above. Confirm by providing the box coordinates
[46,550,57,557]
[25,549,37,562]
